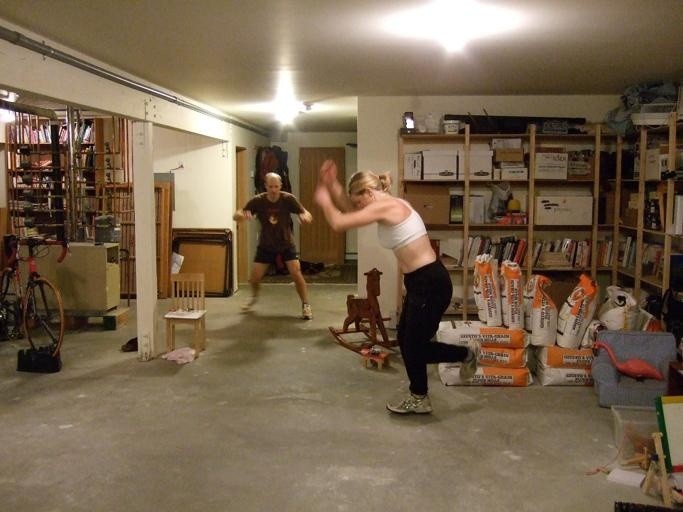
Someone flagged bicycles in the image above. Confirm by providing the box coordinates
[0,231,72,360]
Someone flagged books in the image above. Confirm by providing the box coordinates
[456,234,683,286]
[8,122,92,241]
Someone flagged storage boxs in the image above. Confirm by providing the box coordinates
[402,138,683,235]
[610,404,660,459]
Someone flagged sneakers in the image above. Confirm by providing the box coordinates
[383,393,434,414]
[301,303,313,320]
[458,338,483,385]
[239,294,259,311]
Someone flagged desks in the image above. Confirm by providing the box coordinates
[16,241,121,316]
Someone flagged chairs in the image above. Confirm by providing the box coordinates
[591,330,678,409]
[162,272,208,360]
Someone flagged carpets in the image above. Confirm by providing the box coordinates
[247,258,358,285]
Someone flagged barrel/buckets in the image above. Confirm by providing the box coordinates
[92,212,116,245]
[441,119,462,136]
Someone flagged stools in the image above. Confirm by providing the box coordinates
[360,344,395,373]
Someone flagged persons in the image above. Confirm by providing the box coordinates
[232,172,313,320]
[313,158,482,414]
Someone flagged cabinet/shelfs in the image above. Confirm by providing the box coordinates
[396,111,683,330]
[4,116,131,245]
